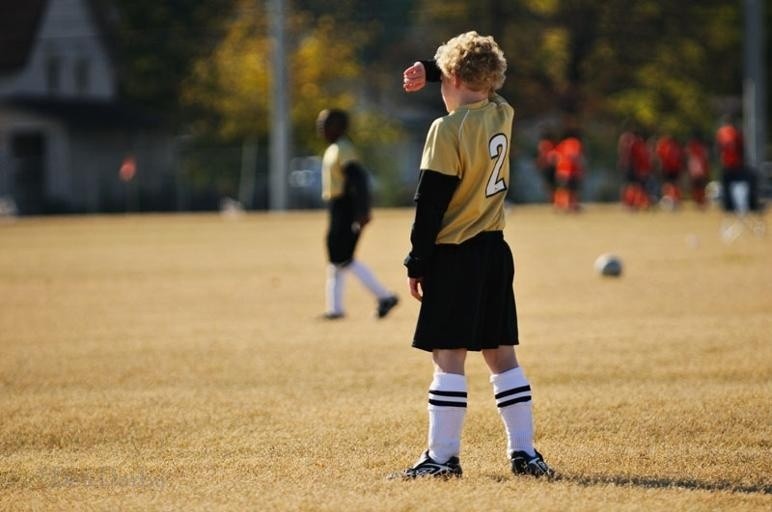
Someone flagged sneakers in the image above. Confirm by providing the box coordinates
[511,448,562,480]
[379,296,397,317]
[386,448,462,481]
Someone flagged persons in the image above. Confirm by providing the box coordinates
[316,108,399,321]
[388,30,563,483]
[538,114,742,215]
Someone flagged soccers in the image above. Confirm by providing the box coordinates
[600,258,621,275]
